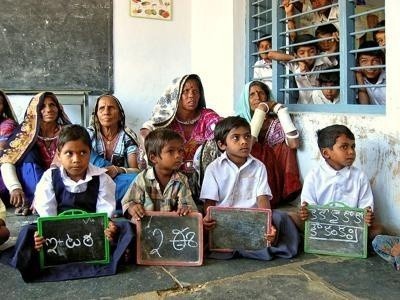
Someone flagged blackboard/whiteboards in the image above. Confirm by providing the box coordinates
[1,0,113,96]
[207,206,271,252]
[137,210,204,266]
[301,206,369,258]
[37,213,110,266]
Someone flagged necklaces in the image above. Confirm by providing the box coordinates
[38,124,61,141]
[174,111,201,125]
[100,131,118,144]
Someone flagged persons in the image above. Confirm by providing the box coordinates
[138,73,226,205]
[85,94,142,205]
[229,80,302,209]
[198,116,300,261]
[286,124,385,243]
[120,127,199,261]
[0,90,72,216]
[252,0,386,105]
[0,89,19,195]
[0,197,11,247]
[8,124,135,284]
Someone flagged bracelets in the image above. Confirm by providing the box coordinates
[258,102,269,114]
[113,166,118,173]
